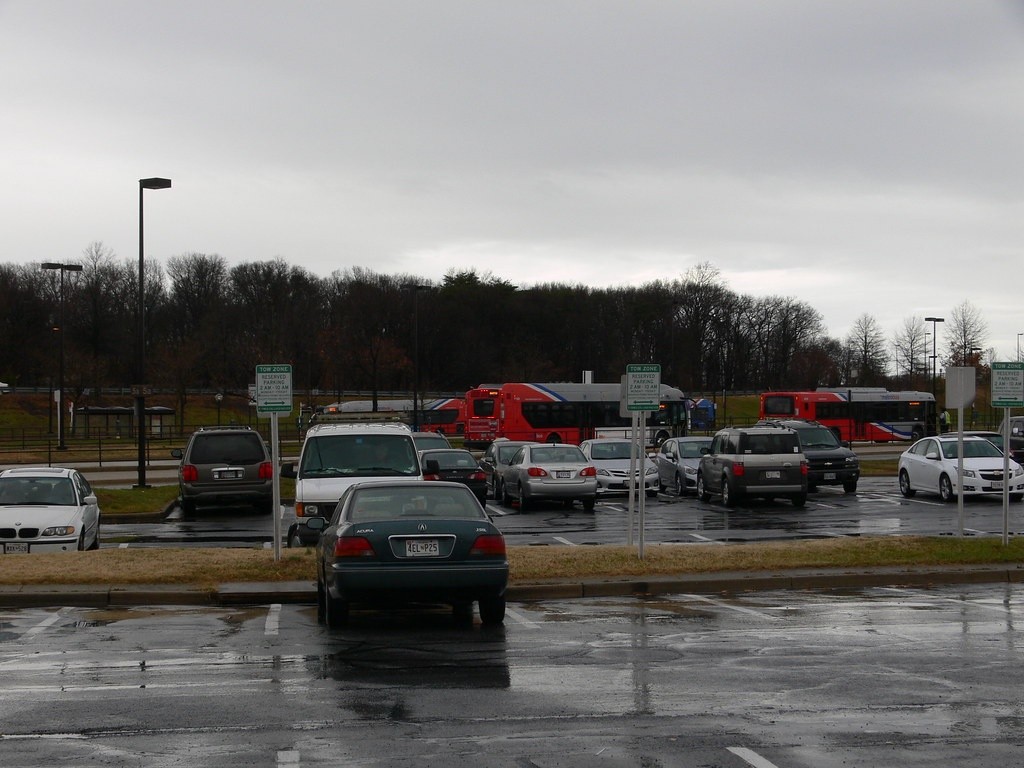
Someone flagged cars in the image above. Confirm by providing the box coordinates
[0,469,101,553]
[282,423,714,627]
[938,416,1024,461]
[897,436,1024,503]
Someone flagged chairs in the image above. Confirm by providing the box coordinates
[363,501,393,517]
[456,459,468,466]
[431,503,467,517]
[535,452,544,461]
[2,479,72,504]
[596,450,607,457]
[564,454,577,463]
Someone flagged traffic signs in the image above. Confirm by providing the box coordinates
[257,365,293,412]
[627,364,660,411]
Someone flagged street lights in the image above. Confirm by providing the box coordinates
[132,176,172,488]
[896,345,900,376]
[928,355,937,393]
[925,318,944,399]
[41,262,83,450]
[1017,333,1023,362]
[925,333,931,378]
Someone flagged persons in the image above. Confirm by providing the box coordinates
[939,407,952,434]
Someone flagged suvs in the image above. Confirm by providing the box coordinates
[696,422,809,508]
[755,418,861,493]
[172,426,273,517]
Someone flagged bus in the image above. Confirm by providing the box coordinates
[465,384,502,449]
[497,383,700,447]
[322,398,465,437]
[761,387,936,444]
